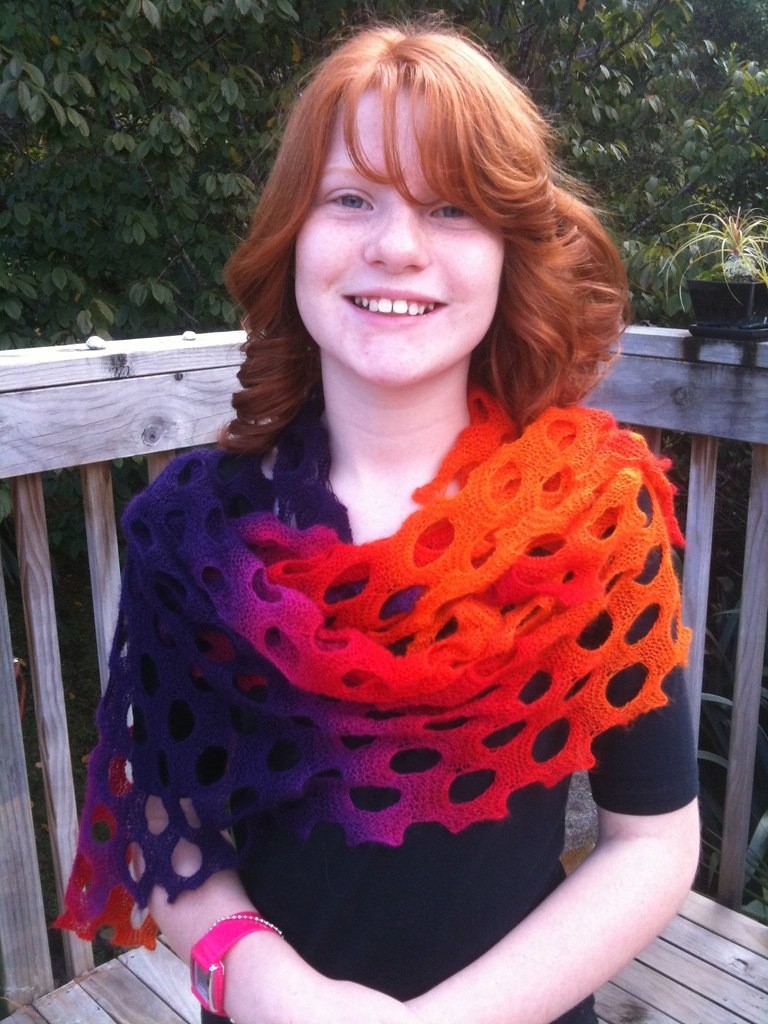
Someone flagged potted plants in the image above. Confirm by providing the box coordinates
[651,198,768,330]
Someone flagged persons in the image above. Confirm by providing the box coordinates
[53,26,700,1024]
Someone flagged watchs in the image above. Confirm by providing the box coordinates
[190,912,281,1017]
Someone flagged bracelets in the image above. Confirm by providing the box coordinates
[207,918,282,940]
